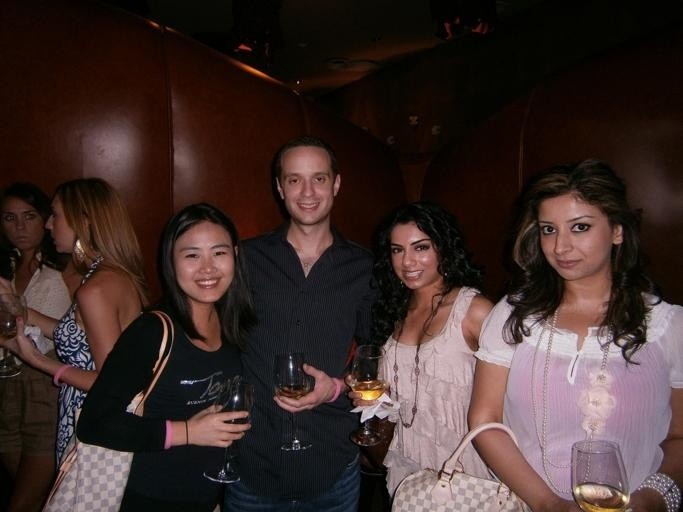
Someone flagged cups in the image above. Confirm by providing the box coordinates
[571,439,631,512]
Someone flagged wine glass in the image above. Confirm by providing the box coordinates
[204,378,255,485]
[272,351,314,453]
[0,291,28,378]
[349,343,391,447]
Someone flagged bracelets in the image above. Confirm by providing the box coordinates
[163,419,170,451]
[53,364,72,386]
[634,472,680,511]
[327,379,340,404]
[183,418,189,445]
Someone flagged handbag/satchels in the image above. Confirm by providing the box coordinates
[41,308,174,512]
[390,422,535,512]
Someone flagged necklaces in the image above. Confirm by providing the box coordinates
[392,286,447,428]
[530,303,613,495]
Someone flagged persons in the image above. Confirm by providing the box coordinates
[75,202,256,512]
[220,132,379,511]
[343,203,494,512]
[0,182,72,511]
[466,160,683,512]
[0,176,149,479]
